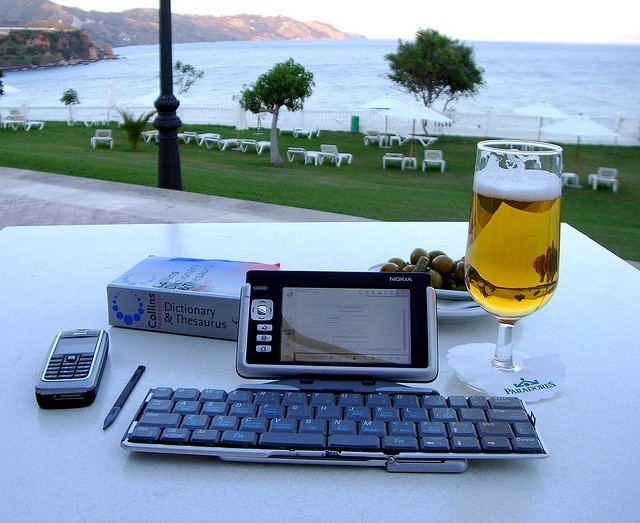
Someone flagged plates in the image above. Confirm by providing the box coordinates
[366,262,490,323]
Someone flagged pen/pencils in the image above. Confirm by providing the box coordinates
[103,365,146,430]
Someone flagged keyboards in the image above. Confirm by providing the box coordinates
[119,385,551,462]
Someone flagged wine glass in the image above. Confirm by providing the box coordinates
[447,139,563,392]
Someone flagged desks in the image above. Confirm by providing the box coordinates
[1,222,640,522]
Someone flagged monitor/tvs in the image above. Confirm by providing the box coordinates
[234,268,440,384]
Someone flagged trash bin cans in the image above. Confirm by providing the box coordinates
[351,115,359,133]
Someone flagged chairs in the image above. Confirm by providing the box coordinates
[364,127,389,145]
[421,149,447,173]
[319,144,353,168]
[382,151,405,172]
[91,127,113,151]
[145,132,269,155]
[277,114,321,139]
[285,145,308,164]
[396,124,438,147]
[8,110,45,132]
[588,165,621,194]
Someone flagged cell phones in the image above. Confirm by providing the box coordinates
[34,327,110,411]
